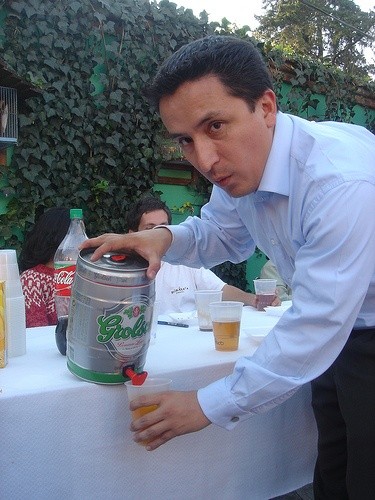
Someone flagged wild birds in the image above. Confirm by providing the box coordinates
[0,99,8,137]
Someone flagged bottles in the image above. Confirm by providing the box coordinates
[53,209,89,356]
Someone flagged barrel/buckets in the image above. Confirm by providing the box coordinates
[66,248,155,385]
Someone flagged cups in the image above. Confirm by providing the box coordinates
[0,250,26,357]
[253,279,277,311]
[208,301,244,352]
[194,290,222,331]
[124,378,172,445]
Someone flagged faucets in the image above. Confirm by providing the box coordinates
[125,370,148,385]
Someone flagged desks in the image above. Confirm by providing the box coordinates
[0,301,318,500]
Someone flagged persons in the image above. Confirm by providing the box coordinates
[261,259,293,301]
[16,205,86,329]
[127,196,282,314]
[78,32,375,500]
